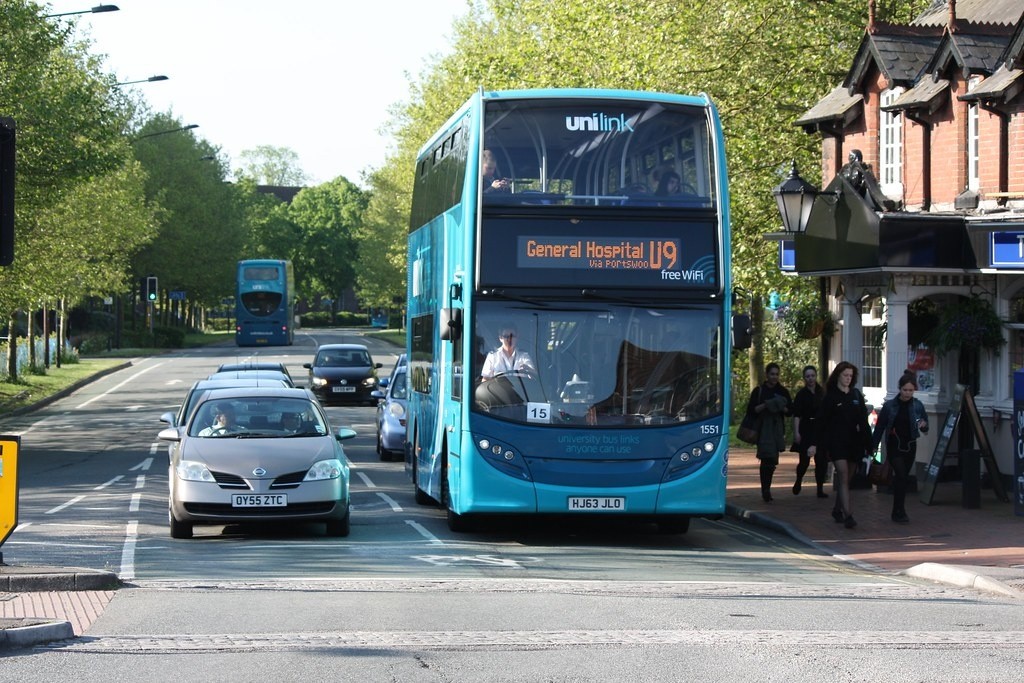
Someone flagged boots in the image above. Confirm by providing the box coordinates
[814,454,829,498]
[793,457,810,495]
[892,497,909,522]
[760,479,774,502]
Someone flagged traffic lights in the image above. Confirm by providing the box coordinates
[147,277,157,301]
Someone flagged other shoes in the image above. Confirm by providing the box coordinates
[831,507,858,529]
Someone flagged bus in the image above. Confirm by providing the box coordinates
[402,85,734,536]
[235,259,295,347]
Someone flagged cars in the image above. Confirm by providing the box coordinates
[379,353,408,390]
[217,363,292,378]
[158,386,359,540]
[370,365,407,462]
[207,370,296,388]
[159,378,291,466]
[303,343,384,408]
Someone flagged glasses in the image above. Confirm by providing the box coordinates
[501,334,517,338]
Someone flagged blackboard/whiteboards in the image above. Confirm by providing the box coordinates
[923,384,1004,486]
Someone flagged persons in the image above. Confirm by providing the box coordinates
[656,173,680,195]
[873,369,929,522]
[808,362,875,529]
[481,322,538,382]
[482,149,512,194]
[748,362,792,502]
[198,403,249,438]
[276,413,308,435]
[790,365,828,497]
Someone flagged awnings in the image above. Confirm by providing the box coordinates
[792,69,1023,134]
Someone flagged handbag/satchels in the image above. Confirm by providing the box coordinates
[736,386,762,443]
